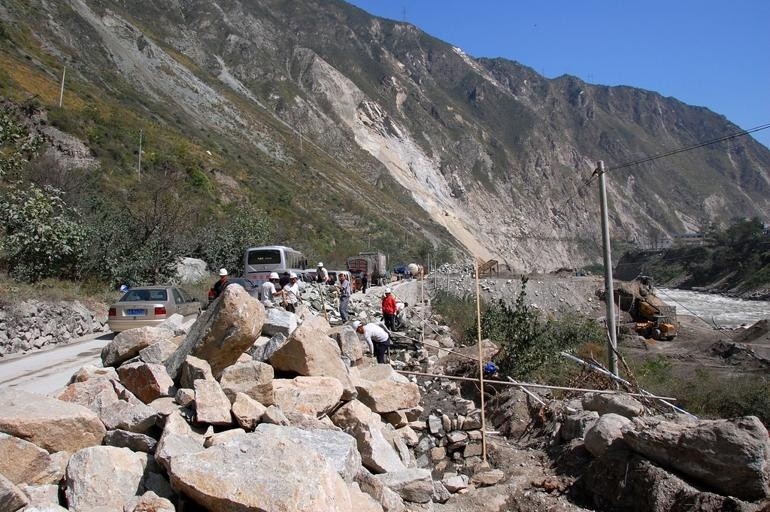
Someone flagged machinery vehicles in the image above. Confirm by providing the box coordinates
[636,314,678,341]
[406,263,423,279]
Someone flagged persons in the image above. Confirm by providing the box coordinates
[278,271,290,290]
[297,272,368,294]
[393,301,408,332]
[282,272,304,313]
[380,287,397,332]
[315,261,330,285]
[352,320,390,364]
[212,268,230,300]
[260,271,283,308]
[334,273,351,326]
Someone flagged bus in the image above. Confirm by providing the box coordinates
[244,245,308,294]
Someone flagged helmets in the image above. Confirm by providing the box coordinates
[385,287,391,294]
[269,271,280,280]
[219,267,228,276]
[289,272,297,279]
[317,261,324,267]
[352,320,363,332]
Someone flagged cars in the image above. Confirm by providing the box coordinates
[108,286,203,332]
[208,278,261,307]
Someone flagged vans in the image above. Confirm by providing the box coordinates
[305,268,352,299]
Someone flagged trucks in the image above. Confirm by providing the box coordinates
[346,250,392,290]
